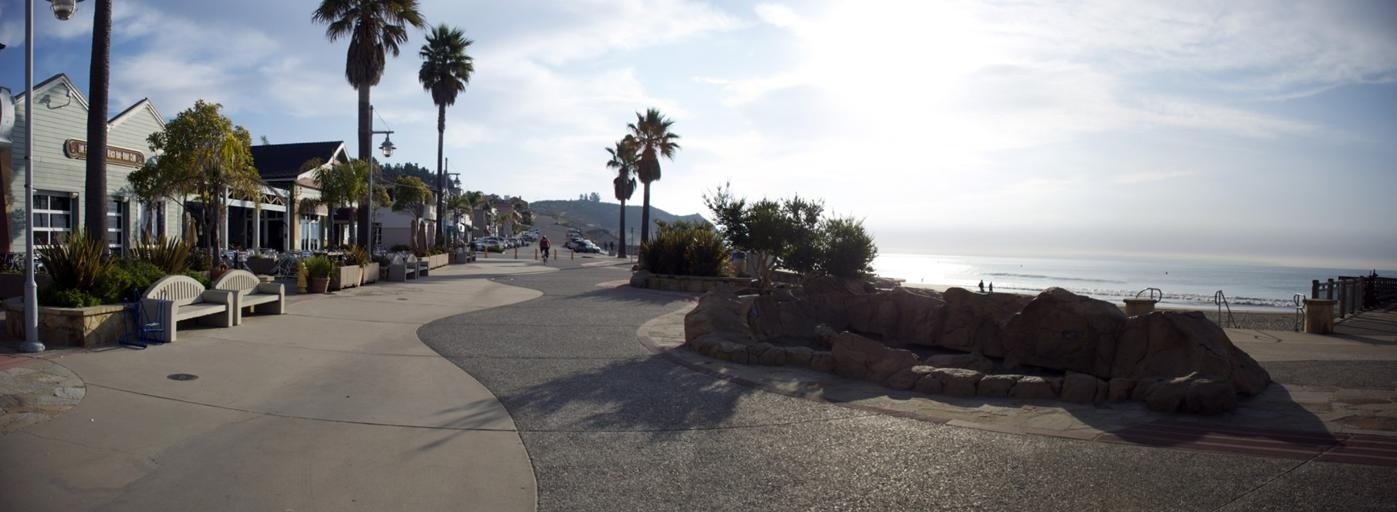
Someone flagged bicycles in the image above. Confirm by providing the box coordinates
[542,248,548,265]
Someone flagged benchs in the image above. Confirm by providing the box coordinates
[455,246,476,264]
[138,268,285,343]
[387,254,428,282]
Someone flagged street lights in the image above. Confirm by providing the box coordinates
[17,0,85,353]
[366,105,396,261]
[444,158,461,249]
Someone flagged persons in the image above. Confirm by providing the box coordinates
[539,235,550,257]
[987,281,992,294]
[978,279,984,291]
[603,240,608,251]
[609,240,614,250]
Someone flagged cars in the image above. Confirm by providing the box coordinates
[471,236,529,254]
[566,227,601,253]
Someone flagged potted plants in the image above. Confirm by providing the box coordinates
[309,253,335,295]
